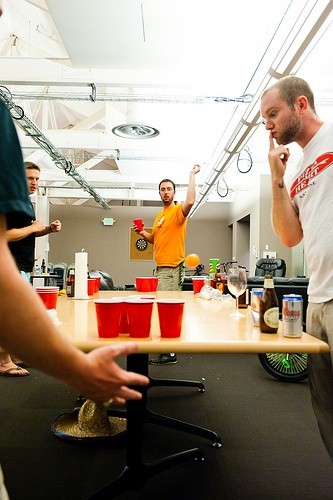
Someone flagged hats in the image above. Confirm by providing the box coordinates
[48,397,128,442]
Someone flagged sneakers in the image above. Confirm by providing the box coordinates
[150,353,177,364]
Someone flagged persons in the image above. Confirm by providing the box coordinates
[134,164,200,365]
[0,99,150,404]
[0,162,62,378]
[261,76,332,461]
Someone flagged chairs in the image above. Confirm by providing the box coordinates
[255,258,286,277]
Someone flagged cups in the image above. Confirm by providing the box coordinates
[90,276,101,293]
[133,218,143,231]
[94,294,155,338]
[157,299,185,338]
[192,278,204,295]
[134,277,159,292]
[35,287,59,316]
[88,279,95,296]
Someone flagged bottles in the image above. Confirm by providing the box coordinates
[35,264,40,276]
[67,268,75,297]
[260,275,279,333]
[238,289,248,309]
[213,272,224,295]
[40,259,47,275]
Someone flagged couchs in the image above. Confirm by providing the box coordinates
[180,276,310,332]
[88,269,124,291]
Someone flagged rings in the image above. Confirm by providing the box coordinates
[101,397,114,406]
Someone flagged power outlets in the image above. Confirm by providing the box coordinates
[263,252,276,259]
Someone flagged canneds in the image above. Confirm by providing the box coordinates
[250,288,262,326]
[282,294,303,339]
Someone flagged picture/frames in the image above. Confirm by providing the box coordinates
[129,227,155,262]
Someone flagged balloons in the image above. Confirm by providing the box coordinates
[185,254,201,269]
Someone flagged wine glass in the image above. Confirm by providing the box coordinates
[227,268,247,318]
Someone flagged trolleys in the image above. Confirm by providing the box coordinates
[247,275,310,382]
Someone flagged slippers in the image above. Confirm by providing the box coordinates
[13,361,32,368]
[0,365,30,376]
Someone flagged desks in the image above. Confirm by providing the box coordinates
[45,290,330,500]
